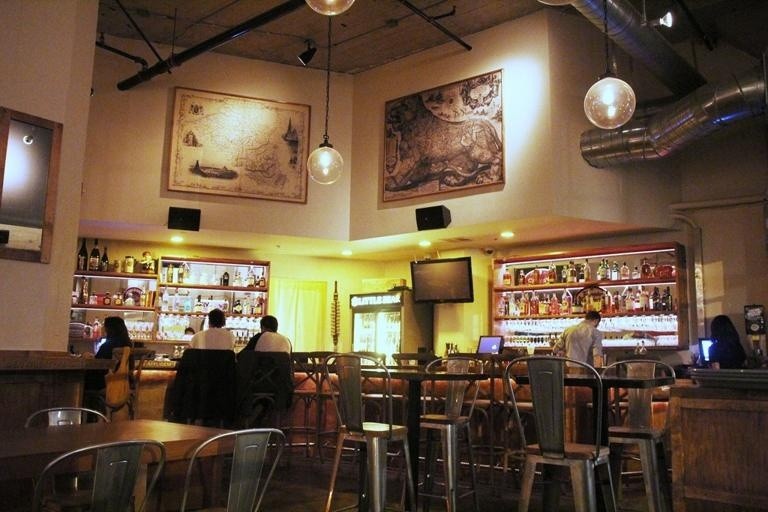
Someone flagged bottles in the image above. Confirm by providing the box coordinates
[443,341,459,357]
[71,237,265,343]
[633,340,647,356]
[495,256,677,314]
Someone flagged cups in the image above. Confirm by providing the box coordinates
[526,345,535,355]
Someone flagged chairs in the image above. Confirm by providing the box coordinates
[182,349,236,429]
[84,347,149,421]
[22,407,287,511]
[236,351,290,429]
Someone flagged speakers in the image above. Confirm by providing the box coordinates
[415,205,451,231]
[168,207,201,231]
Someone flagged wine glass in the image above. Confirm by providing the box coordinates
[500,315,678,348]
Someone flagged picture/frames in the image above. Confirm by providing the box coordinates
[166,85,311,205]
[382,69,505,205]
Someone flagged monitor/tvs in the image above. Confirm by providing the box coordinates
[410,256,474,303]
[476,336,504,354]
[698,337,717,364]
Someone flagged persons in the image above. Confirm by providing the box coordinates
[81,314,133,426]
[707,313,747,370]
[188,307,235,354]
[240,314,293,356]
[551,310,604,368]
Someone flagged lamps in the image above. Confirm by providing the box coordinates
[305,0,356,186]
[297,36,316,67]
[584,1,637,131]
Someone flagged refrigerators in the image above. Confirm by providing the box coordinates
[349,286,434,365]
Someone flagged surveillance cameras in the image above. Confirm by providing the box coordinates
[485,247,494,254]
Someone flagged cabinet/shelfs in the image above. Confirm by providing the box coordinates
[67,270,159,345]
[156,255,271,348]
[488,241,690,350]
[668,384,767,512]
